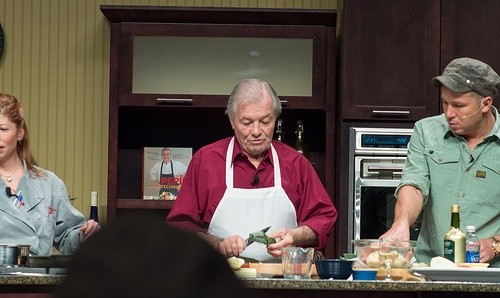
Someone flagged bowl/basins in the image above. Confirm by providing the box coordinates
[27,255,52,269]
[51,254,72,267]
[314,259,355,280]
[353,239,418,269]
[352,269,377,280]
[0,244,30,266]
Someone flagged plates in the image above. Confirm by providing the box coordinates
[410,267,500,284]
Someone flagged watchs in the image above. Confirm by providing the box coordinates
[489,237,500,256]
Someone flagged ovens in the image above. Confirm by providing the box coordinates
[348,126,423,255]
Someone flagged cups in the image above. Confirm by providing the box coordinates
[281,247,314,280]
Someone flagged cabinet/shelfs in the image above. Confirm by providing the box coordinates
[338,0,500,124]
[99,4,337,259]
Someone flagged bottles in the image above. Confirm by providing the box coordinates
[89,191,99,223]
[465,225,483,263]
[443,204,465,264]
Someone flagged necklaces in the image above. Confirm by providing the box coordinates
[2,162,21,183]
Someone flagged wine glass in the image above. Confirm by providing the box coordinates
[379,237,400,282]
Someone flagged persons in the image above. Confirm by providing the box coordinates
[0,93,101,257]
[166,78,337,262]
[380,57,500,264]
[150,148,187,196]
[61,219,246,298]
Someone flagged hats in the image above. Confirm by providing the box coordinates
[431,57,500,99]
